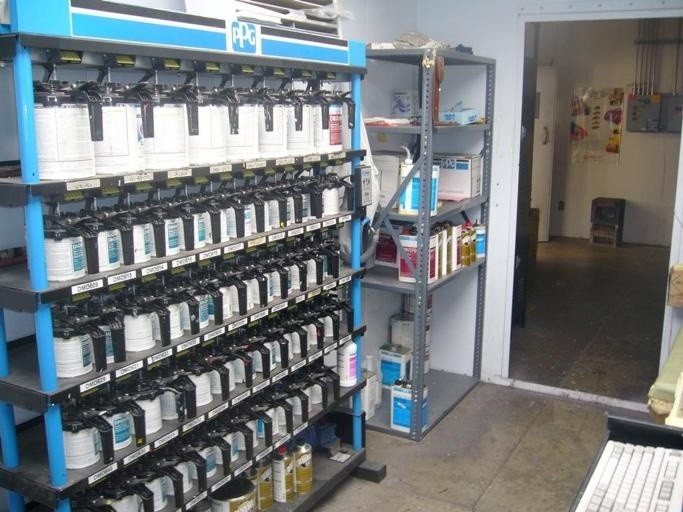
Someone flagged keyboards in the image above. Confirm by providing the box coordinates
[575,440,682,512]
